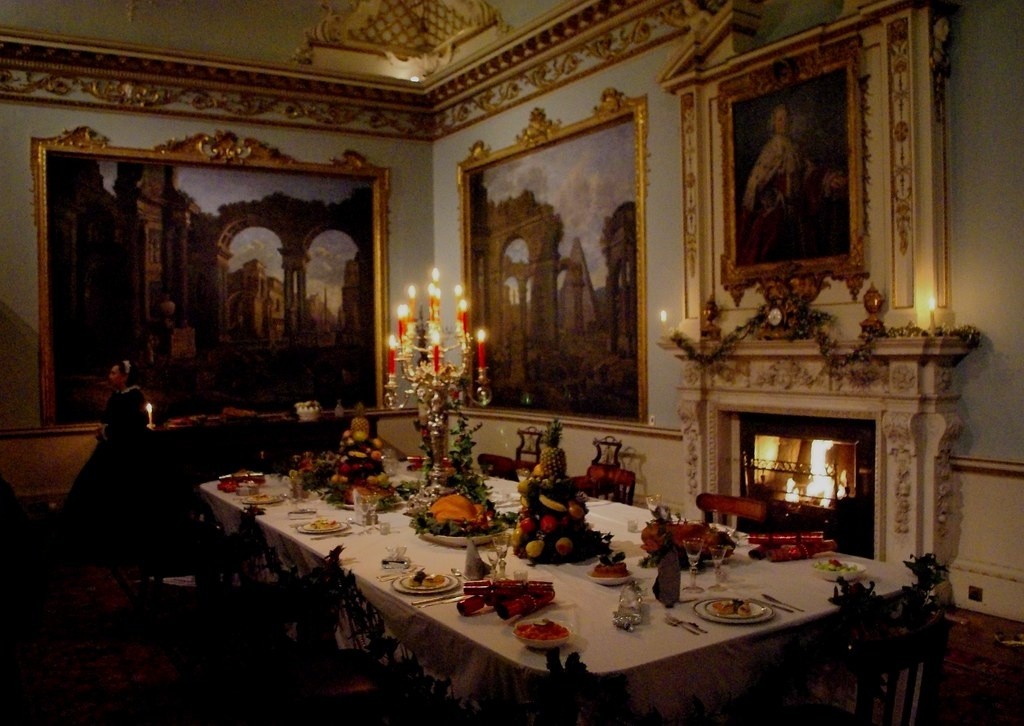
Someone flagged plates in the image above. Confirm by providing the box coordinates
[393,574,458,594]
[693,597,775,623]
[242,498,284,504]
[423,532,502,547]
[513,619,569,650]
[297,522,347,533]
[587,572,633,585]
[814,560,866,582]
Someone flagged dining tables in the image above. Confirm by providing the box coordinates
[198,470,931,726]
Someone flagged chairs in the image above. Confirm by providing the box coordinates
[125,452,769,726]
[768,615,951,726]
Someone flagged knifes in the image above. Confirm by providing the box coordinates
[762,594,805,612]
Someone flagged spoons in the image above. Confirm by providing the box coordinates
[451,567,470,582]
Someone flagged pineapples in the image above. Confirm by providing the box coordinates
[349,401,369,436]
[539,417,567,478]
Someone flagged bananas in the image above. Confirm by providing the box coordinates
[537,493,568,513]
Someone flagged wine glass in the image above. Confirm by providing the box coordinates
[355,496,372,536]
[290,478,307,503]
[487,547,501,581]
[708,546,733,591]
[492,533,511,580]
[366,495,380,534]
[681,538,704,594]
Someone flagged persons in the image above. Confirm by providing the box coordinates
[0,476,46,653]
[58,359,189,557]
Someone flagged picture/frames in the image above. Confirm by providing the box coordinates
[27,120,389,428]
[713,35,872,304]
[456,84,652,428]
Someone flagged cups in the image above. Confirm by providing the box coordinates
[628,519,638,532]
[382,458,398,475]
[380,523,390,535]
[514,570,528,581]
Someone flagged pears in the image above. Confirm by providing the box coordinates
[526,540,545,557]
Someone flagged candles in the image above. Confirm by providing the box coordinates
[386,266,489,373]
[659,310,668,336]
[929,296,937,331]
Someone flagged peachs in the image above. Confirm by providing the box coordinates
[556,537,573,555]
